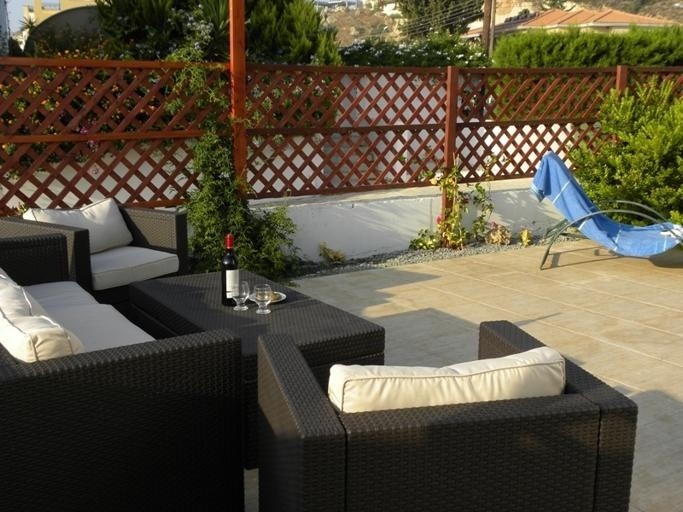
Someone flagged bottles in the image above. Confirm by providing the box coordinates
[221,233,240,307]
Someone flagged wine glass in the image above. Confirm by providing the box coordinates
[232,280,250,311]
[253,284,272,314]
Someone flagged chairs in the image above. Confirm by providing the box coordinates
[531,151,683,270]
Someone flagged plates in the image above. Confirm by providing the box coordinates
[249,291,287,303]
